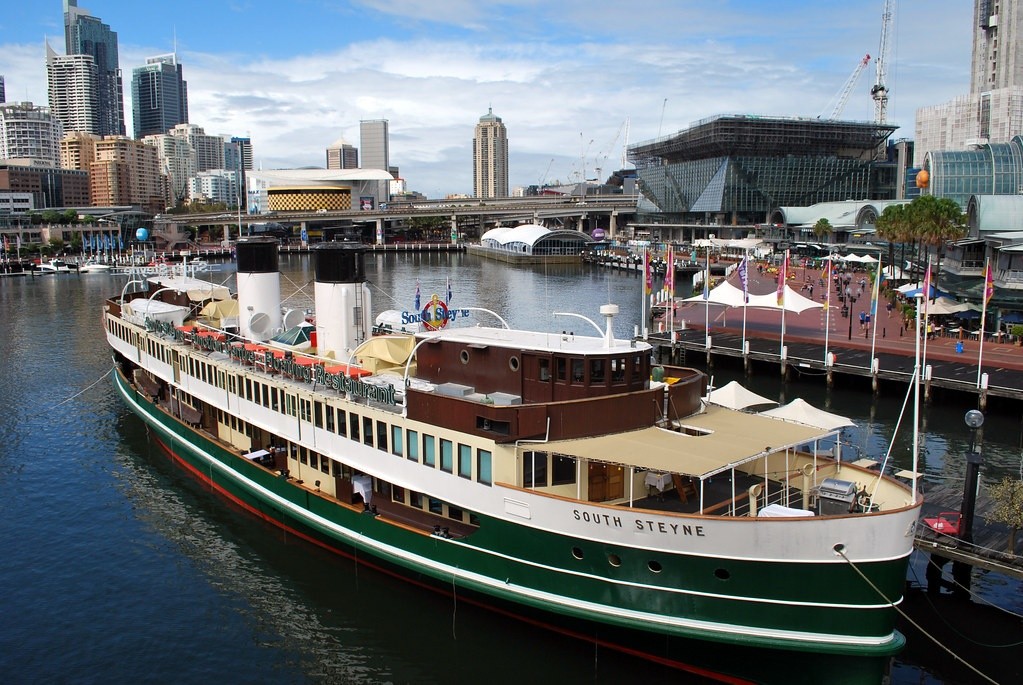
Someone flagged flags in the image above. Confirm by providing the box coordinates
[642,247,654,295]
[702,254,711,299]
[738,255,749,303]
[776,254,791,304]
[980,264,994,325]
[821,260,836,310]
[922,264,932,320]
[415,282,420,310]
[446,284,452,306]
[869,262,887,316]
[663,246,675,296]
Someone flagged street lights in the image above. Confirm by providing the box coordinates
[841,272,852,318]
[845,287,862,340]
[834,262,849,301]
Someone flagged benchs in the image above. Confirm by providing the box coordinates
[135,371,160,402]
[165,393,202,428]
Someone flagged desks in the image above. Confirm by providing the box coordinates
[949,328,965,340]
[253,350,296,372]
[645,472,675,502]
[276,356,325,381]
[934,326,945,337]
[323,366,372,382]
[353,474,372,503]
[970,331,986,340]
[176,327,202,342]
[190,332,227,351]
[992,332,1007,344]
[229,344,269,365]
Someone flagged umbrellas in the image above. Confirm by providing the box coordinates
[997,312,1023,323]
[815,252,880,268]
[895,280,986,329]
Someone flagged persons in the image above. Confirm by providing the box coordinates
[846,287,852,298]
[886,301,892,319]
[861,279,865,291]
[756,264,762,272]
[927,321,936,340]
[954,340,964,353]
[856,287,862,297]
[834,262,848,271]
[858,310,871,329]
[801,275,824,299]
[843,279,849,288]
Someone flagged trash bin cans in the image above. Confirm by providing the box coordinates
[842,308,848,317]
[955,342,963,353]
[839,295,843,301]
[834,277,839,283]
[836,286,840,291]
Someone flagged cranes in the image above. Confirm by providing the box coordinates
[816,51,872,122]
[534,97,669,204]
[870,0,900,159]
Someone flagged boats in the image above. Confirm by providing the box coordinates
[101,236,925,685]
[36,258,79,273]
[78,255,115,274]
